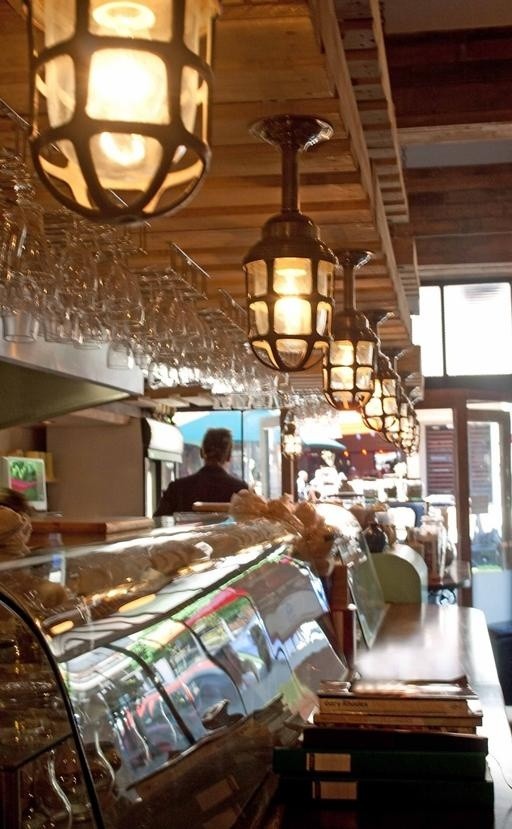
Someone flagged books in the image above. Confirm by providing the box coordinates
[30,514,153,534]
[311,676,484,736]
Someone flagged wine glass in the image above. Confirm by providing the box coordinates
[0,100,305,409]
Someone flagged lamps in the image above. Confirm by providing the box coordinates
[239,112,339,373]
[376,348,423,457]
[21,0,224,227]
[321,248,381,411]
[359,309,401,431]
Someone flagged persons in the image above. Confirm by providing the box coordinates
[152,427,258,518]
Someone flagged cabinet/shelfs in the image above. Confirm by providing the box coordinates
[27,515,153,541]
[303,600,512,829]
[1,511,356,829]
